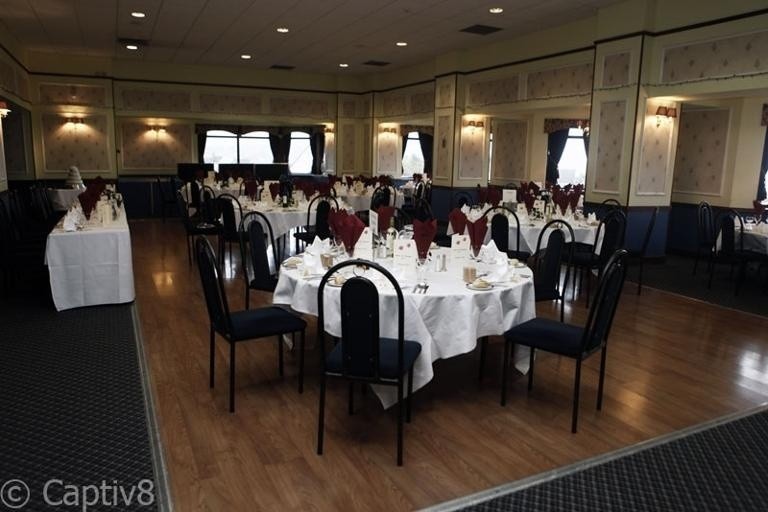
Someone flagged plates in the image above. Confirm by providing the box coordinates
[328,280,347,287]
[513,263,527,267]
[469,284,494,290]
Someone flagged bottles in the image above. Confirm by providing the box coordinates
[435,255,441,271]
[442,255,447,271]
[282,185,288,208]
[386,217,399,257]
[545,205,550,223]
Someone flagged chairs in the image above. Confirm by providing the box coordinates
[693,201,768,298]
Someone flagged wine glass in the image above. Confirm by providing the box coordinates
[416,258,429,286]
[575,207,583,224]
